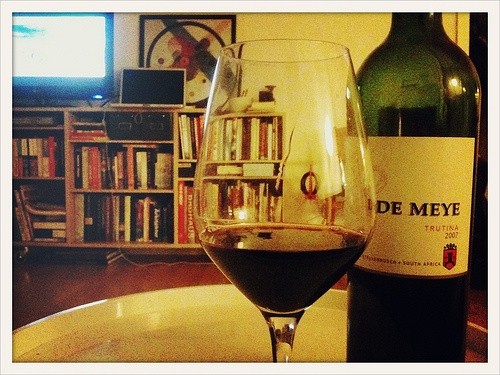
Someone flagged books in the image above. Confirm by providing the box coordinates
[73,193,174,244]
[179,163,277,177]
[14,184,67,243]
[74,144,175,191]
[178,180,282,244]
[178,112,282,160]
[69,114,109,141]
[12,136,65,179]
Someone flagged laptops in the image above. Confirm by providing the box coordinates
[109,69,187,109]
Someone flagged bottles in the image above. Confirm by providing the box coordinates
[344,12,483,363]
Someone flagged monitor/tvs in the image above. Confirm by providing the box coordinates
[11,11,114,107]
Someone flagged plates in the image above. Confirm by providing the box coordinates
[12,283,487,363]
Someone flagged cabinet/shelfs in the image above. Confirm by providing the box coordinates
[12,107,286,255]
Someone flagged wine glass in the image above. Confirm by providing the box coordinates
[191,36,378,363]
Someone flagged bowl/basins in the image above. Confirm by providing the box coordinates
[230,96,254,112]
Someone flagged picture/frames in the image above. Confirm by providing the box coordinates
[140,15,236,107]
